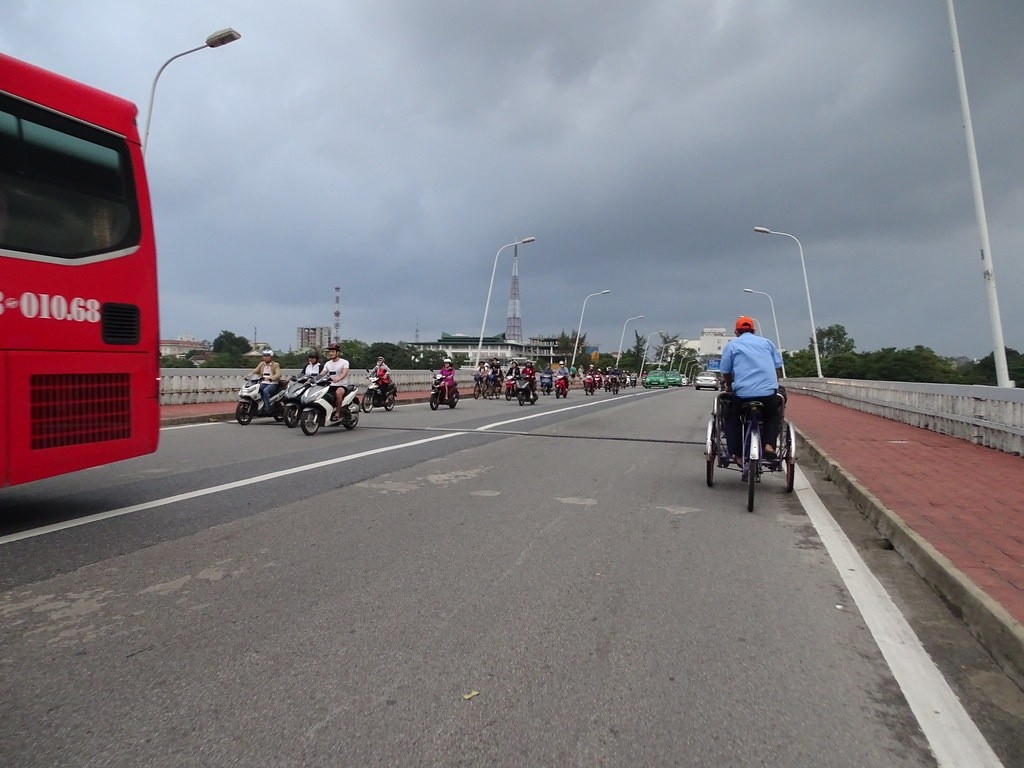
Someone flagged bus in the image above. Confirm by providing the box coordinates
[0,52,162,486]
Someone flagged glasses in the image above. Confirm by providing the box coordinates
[263,353,270,356]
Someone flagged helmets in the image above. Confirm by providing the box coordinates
[444,359,452,365]
[263,349,274,356]
[479,358,618,372]
[377,356,385,365]
[308,353,318,358]
[326,343,340,350]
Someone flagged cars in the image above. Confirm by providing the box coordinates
[644,368,669,389]
[695,371,720,391]
[680,374,689,386]
[665,369,683,387]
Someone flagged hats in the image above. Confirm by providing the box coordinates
[735,316,755,334]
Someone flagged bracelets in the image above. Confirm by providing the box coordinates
[726,386,731,388]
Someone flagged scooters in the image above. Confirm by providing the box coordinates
[235,372,290,425]
[299,370,363,436]
[584,372,646,396]
[428,368,460,410]
[539,371,555,396]
[279,373,319,428]
[504,370,538,406]
[553,372,569,399]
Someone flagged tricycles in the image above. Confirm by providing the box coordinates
[703,384,795,513]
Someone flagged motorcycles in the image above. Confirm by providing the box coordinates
[361,368,398,413]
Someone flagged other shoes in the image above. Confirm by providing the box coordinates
[763,450,777,459]
[333,413,340,420]
[728,454,737,462]
[385,398,388,403]
[530,393,533,398]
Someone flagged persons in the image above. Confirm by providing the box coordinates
[521,361,537,398]
[301,351,323,375]
[583,364,597,389]
[556,361,570,390]
[621,370,637,386]
[577,364,584,385]
[543,363,553,389]
[440,358,454,400]
[316,342,349,420]
[489,358,504,393]
[366,357,391,403]
[720,318,784,458]
[570,365,577,385]
[604,365,622,391]
[642,371,648,377]
[473,361,488,390]
[597,368,603,385]
[243,349,280,414]
[506,361,520,388]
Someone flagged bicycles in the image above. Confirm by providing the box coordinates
[472,373,502,400]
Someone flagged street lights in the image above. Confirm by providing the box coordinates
[659,343,675,369]
[639,331,664,377]
[753,226,826,379]
[140,28,242,160]
[670,350,708,382]
[476,236,536,370]
[571,290,610,367]
[616,316,644,368]
[740,289,787,379]
